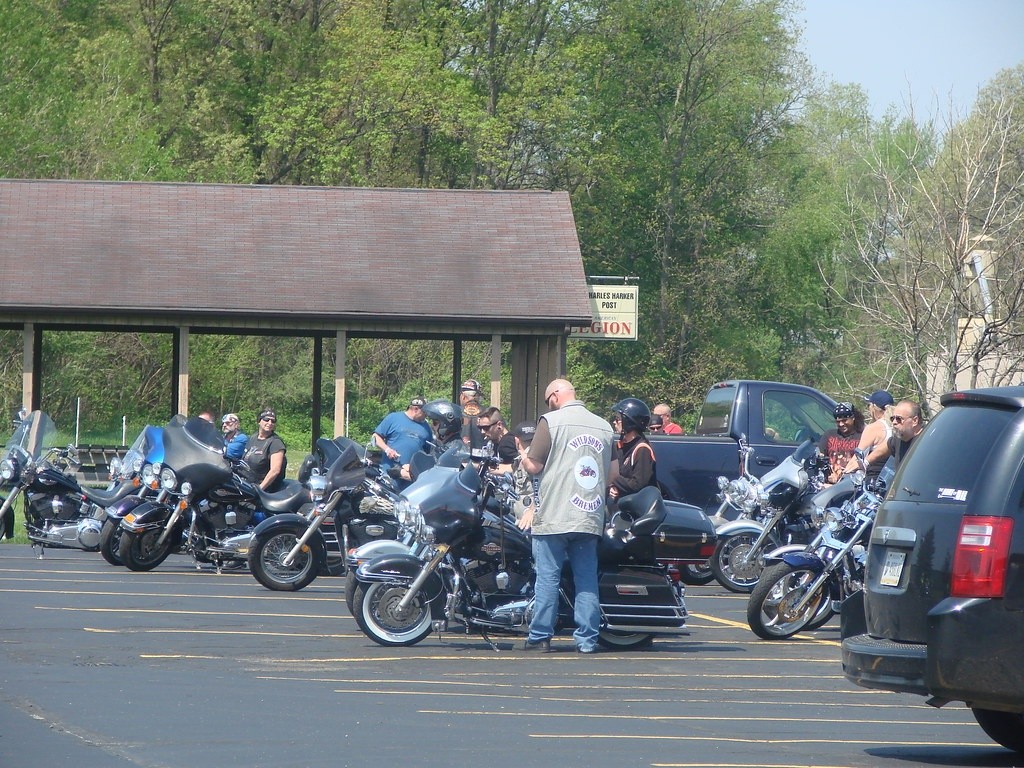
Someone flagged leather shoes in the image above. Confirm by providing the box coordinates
[219,560,246,570]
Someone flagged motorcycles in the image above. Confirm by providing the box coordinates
[0,408,899,650]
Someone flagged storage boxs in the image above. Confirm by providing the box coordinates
[645,499,718,565]
[298,455,318,484]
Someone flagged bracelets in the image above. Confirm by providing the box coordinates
[863,458,872,467]
[384,447,390,453]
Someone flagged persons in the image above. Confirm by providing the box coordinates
[827,402,868,483]
[222,408,287,570]
[653,402,683,434]
[197,410,217,425]
[220,413,248,462]
[846,390,895,476]
[511,377,614,656]
[370,379,538,533]
[646,414,665,434]
[609,397,662,504]
[842,399,924,490]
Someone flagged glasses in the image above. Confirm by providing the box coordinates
[479,421,498,431]
[614,415,621,421]
[263,418,276,423]
[890,416,904,423]
[545,390,558,405]
[650,426,661,430]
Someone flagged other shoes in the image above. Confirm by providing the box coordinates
[512,640,550,653]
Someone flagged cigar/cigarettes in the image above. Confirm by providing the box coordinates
[484,436,488,440]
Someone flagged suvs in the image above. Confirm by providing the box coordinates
[839,385,1024,756]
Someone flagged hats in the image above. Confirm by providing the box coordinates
[649,414,663,426]
[513,421,536,441]
[865,391,894,411]
[221,414,238,425]
[833,402,855,418]
[257,409,276,423]
[461,379,480,391]
[411,397,426,406]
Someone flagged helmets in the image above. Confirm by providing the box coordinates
[611,398,651,431]
[421,400,464,437]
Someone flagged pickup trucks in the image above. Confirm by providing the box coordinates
[606,379,869,521]
